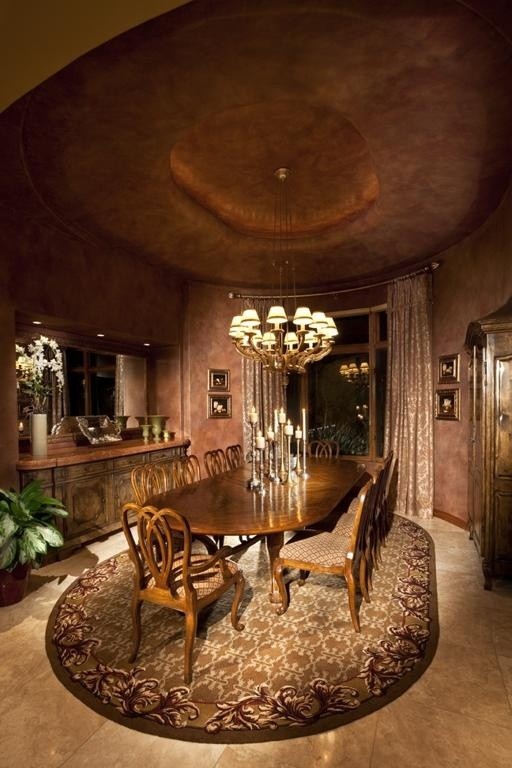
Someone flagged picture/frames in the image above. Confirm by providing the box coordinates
[436,350,463,385]
[434,386,462,423]
[206,364,231,391]
[201,390,233,419]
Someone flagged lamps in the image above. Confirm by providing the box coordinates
[229,163,341,376]
[338,360,371,389]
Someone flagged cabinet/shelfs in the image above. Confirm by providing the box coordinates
[73,460,109,550]
[110,446,183,534]
[19,464,74,552]
[461,294,512,592]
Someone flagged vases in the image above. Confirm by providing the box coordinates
[112,413,145,428]
[28,411,50,458]
[139,414,171,443]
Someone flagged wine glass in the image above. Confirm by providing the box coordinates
[147,415,168,441]
[115,415,130,429]
[163,430,169,441]
[139,424,153,441]
[135,416,146,428]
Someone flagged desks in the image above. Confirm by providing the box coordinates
[140,454,366,618]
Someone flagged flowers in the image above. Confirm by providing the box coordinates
[12,334,69,412]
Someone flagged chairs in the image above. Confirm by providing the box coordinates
[119,500,248,689]
[126,441,259,551]
[274,446,405,630]
[308,437,343,463]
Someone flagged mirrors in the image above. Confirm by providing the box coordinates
[14,324,153,448]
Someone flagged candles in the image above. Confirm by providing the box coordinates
[243,400,313,447]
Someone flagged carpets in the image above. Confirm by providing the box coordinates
[42,508,442,743]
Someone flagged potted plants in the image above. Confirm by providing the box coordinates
[0,475,70,607]
